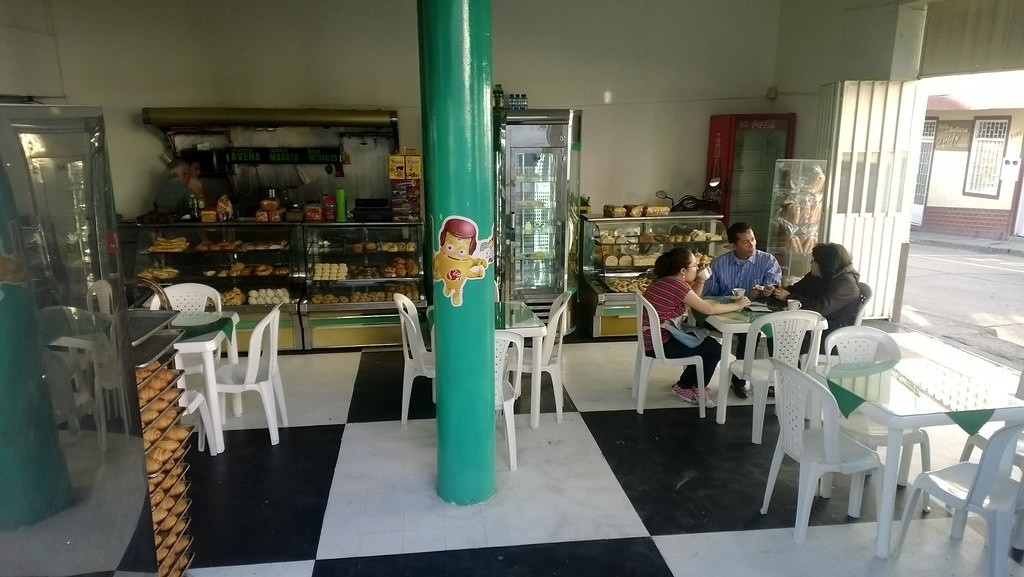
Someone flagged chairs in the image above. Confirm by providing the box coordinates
[151,284,289,456]
[394,279,573,472]
[36,280,132,455]
[632,282,1023,577]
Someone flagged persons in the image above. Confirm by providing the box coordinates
[701,222,783,399]
[642,247,751,408]
[150,159,211,247]
[187,160,210,211]
[764,242,862,398]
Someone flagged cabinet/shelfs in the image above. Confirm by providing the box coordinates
[128,213,427,350]
[580,211,724,342]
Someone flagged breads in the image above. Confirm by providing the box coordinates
[133,359,190,577]
[603,203,671,217]
[139,197,418,305]
[784,173,826,254]
[593,228,722,293]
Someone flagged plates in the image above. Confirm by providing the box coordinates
[784,307,803,311]
[730,296,740,300]
[704,300,720,304]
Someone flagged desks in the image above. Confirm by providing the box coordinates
[170,310,242,454]
[426,300,548,428]
[688,295,828,424]
[36,307,113,412]
[809,358,1024,560]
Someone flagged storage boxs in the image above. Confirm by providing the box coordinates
[388,153,423,188]
[202,207,218,222]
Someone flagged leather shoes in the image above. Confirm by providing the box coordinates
[732,383,749,398]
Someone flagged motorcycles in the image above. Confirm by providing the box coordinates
[656,176,722,252]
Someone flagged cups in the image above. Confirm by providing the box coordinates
[787,299,801,310]
[697,268,710,280]
[732,288,746,299]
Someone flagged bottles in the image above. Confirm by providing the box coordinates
[492,84,504,110]
[324,198,335,220]
[521,94,528,109]
[514,94,521,109]
[304,200,322,221]
[707,133,721,209]
[189,194,199,222]
[508,94,514,109]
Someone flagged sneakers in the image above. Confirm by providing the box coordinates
[694,386,715,409]
[672,383,697,404]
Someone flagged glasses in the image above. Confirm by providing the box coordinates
[686,264,697,269]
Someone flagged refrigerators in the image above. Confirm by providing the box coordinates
[493,108,582,337]
[706,112,797,275]
